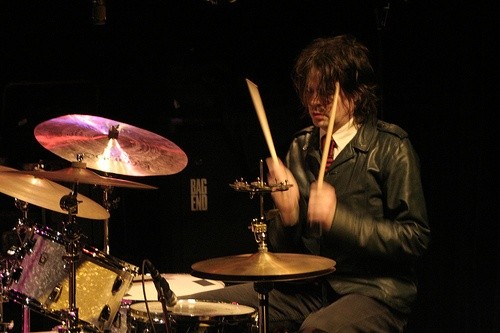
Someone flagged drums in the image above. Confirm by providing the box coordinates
[110,271,260,333]
[7,225,139,333]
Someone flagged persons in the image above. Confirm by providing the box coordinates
[265,36,432,333]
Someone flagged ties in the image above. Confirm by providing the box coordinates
[319,138,336,170]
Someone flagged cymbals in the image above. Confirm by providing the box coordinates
[33,114,189,176]
[0,165,111,220]
[192,252,336,281]
[33,167,156,190]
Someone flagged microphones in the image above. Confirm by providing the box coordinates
[144,259,178,311]
